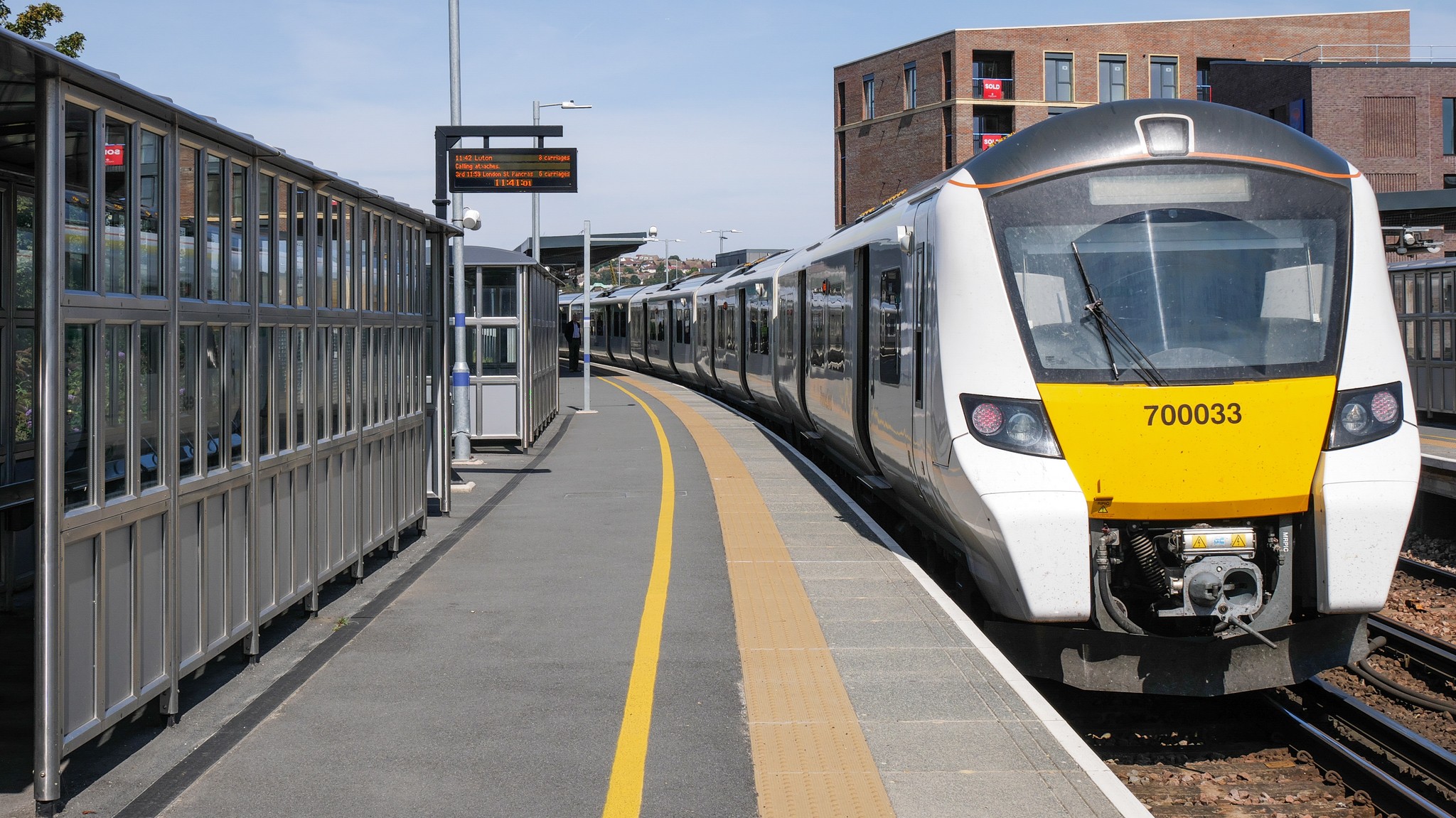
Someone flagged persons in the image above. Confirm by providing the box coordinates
[564,314,582,372]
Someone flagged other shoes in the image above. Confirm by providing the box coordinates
[570,369,574,372]
[574,369,581,372]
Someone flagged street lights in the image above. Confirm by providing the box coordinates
[700,230,742,254]
[619,254,632,285]
[668,259,677,278]
[533,99,592,264]
[653,239,686,282]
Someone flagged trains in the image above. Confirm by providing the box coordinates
[558,99,1422,697]
[8,183,416,459]
